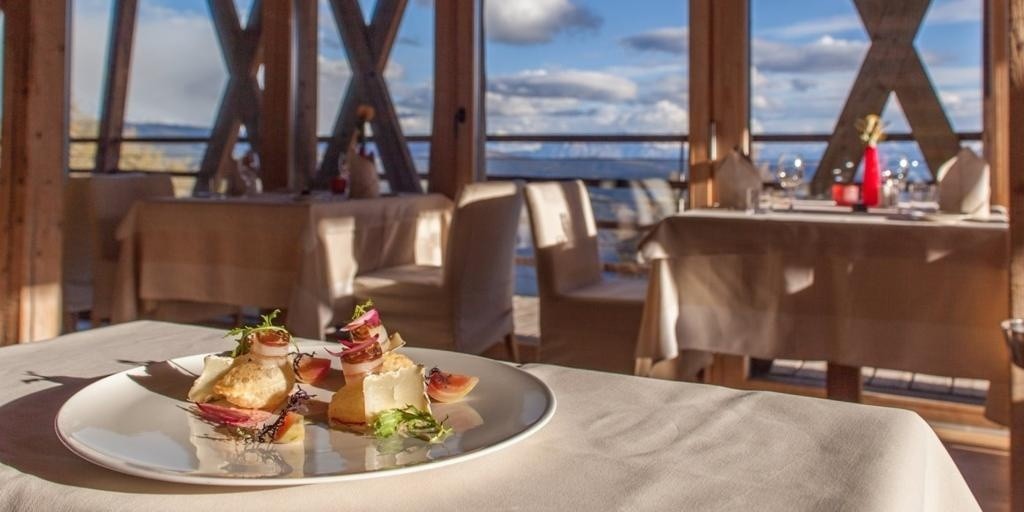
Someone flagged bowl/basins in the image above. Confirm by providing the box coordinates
[829,179,862,206]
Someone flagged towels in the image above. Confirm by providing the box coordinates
[349,148,380,198]
[714,148,761,210]
[936,146,991,215]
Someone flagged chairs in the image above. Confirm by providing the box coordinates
[347,179,519,352]
[523,178,655,373]
[63,167,237,324]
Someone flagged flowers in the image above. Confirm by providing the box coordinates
[352,106,376,154]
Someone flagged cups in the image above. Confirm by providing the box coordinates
[909,182,941,220]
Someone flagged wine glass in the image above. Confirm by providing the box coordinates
[881,153,909,209]
[777,149,805,215]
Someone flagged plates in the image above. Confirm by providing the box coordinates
[55,343,557,492]
